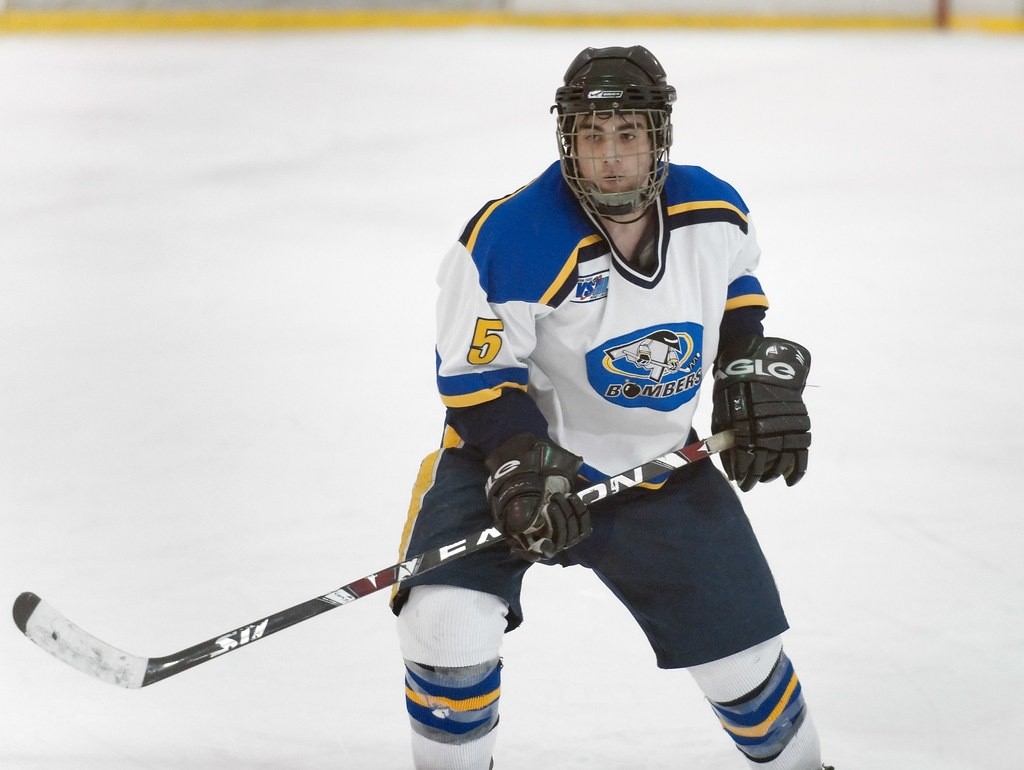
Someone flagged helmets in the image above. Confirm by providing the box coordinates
[551,45,676,217]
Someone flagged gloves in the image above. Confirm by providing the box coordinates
[480,431,594,563]
[709,336,812,492]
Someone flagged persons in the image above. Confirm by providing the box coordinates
[391,43,822,770]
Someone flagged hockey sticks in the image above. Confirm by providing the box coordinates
[11,428,736,691]
